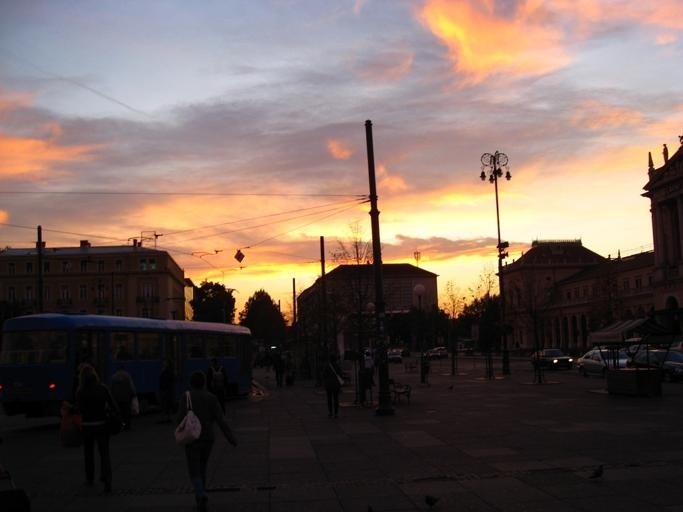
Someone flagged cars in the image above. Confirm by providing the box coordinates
[528,340,682,385]
[363,345,447,361]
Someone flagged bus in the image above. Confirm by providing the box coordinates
[0,313,255,418]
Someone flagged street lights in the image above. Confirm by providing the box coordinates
[365,301,375,371]
[479,149,515,376]
[411,282,427,386]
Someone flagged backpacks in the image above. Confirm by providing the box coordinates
[210,363,225,386]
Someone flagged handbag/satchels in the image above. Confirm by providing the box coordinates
[131,395,139,415]
[174,413,203,443]
[337,375,344,384]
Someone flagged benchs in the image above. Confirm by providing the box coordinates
[391,384,412,405]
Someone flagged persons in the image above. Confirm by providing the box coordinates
[325,357,346,418]
[274,353,285,387]
[71,364,136,493]
[175,373,238,511]
